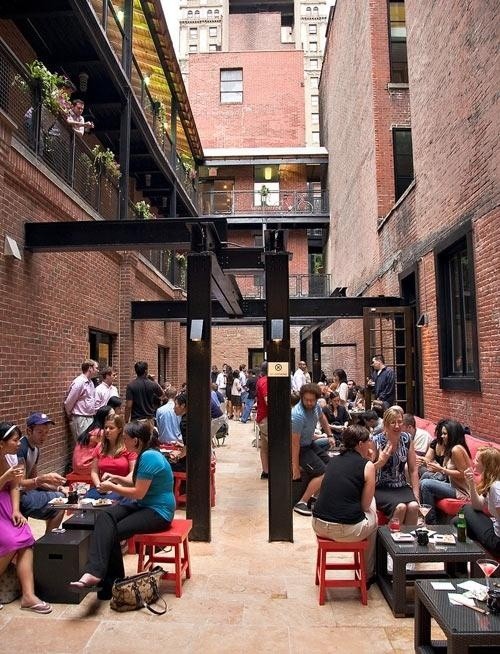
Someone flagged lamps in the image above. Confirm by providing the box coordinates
[189,318,204,342]
[412,255,430,329]
[270,316,284,344]
[2,233,22,263]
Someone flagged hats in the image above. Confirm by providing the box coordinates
[26,413,56,427]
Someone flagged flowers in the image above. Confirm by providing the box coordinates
[10,57,76,160]
[77,142,158,220]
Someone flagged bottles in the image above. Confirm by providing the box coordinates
[456,510,468,542]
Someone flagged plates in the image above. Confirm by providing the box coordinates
[391,532,415,542]
[48,497,69,504]
[92,498,113,506]
[433,534,456,544]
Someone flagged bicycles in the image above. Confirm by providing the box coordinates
[271,191,313,216]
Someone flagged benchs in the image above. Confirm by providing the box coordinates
[413,416,500,516]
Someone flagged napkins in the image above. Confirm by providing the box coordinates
[431,582,455,590]
[457,579,490,593]
[449,592,476,606]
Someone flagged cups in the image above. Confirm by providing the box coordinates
[67,491,78,503]
[415,529,430,545]
[389,517,401,531]
[490,590,500,612]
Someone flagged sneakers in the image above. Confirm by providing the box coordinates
[294,502,312,515]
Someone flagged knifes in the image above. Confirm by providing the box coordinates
[451,598,490,616]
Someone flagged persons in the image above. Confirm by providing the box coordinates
[288,355,499,588]
[2,354,188,612]
[45,99,94,185]
[24,79,77,158]
[209,362,269,480]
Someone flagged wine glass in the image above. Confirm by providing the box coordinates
[418,503,433,528]
[475,558,499,587]
[13,456,26,490]
[72,481,86,505]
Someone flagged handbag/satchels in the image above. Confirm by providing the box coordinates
[110,566,169,614]
[0,563,22,604]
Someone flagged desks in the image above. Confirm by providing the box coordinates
[375,525,486,618]
[63,513,94,530]
[71,497,114,514]
[414,577,499,653]
[32,530,92,605]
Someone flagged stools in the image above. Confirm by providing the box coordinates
[171,467,216,507]
[375,509,389,526]
[214,431,227,446]
[129,518,194,600]
[66,471,96,517]
[312,535,368,607]
[290,464,312,510]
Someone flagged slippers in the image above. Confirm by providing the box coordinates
[20,601,53,613]
[68,580,104,592]
